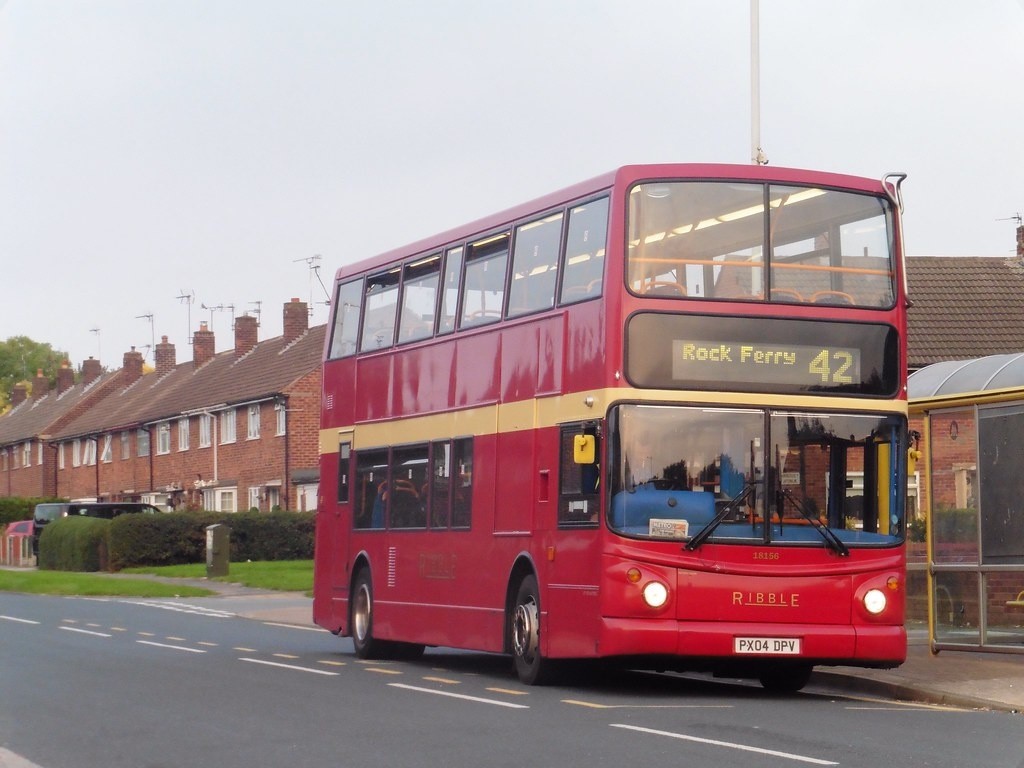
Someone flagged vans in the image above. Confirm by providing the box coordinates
[32,502,165,568]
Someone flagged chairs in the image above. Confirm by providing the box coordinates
[339,285,857,360]
[359,471,472,529]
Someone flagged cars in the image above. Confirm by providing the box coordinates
[3,520,36,539]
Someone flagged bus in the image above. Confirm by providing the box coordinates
[312,162,924,696]
